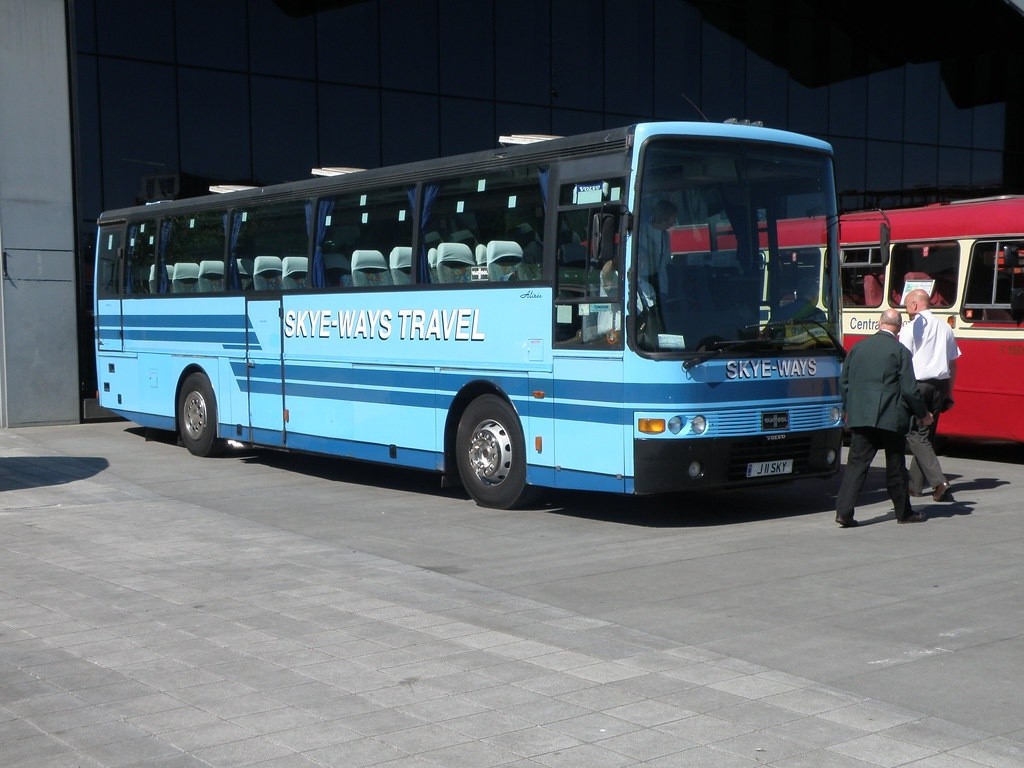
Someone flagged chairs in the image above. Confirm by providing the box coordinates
[147,224,585,286]
[904,272,945,307]
[863,275,883,308]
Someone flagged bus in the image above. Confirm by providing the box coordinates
[576,194,1024,446]
[94,121,896,509]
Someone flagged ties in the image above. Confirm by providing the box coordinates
[637,288,647,307]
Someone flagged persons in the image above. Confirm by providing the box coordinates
[597,247,657,335]
[985,255,1024,320]
[899,290,961,501]
[762,278,827,338]
[639,200,678,299]
[835,309,928,527]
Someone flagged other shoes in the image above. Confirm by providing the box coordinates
[897,511,929,523]
[932,480,953,502]
[835,515,859,527]
[909,490,922,498]
